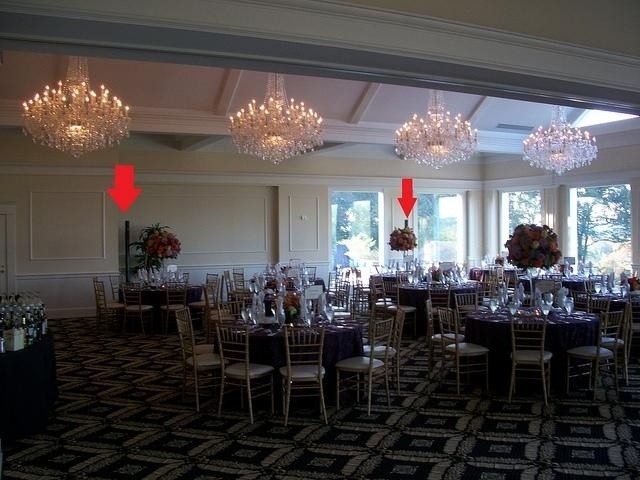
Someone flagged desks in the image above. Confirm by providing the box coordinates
[1,325,59,446]
[464,306,603,398]
[207,310,366,422]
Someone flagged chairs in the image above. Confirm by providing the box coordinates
[362,306,407,400]
[331,315,396,417]
[218,262,638,343]
[599,300,632,389]
[435,305,492,396]
[212,323,277,426]
[563,309,624,403]
[424,298,466,381]
[505,311,554,407]
[159,281,191,336]
[94,261,218,328]
[275,325,331,427]
[175,306,230,411]
[119,281,155,339]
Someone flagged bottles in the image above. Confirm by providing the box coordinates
[0,303,49,354]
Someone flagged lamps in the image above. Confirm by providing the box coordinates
[392,88,482,171]
[18,55,132,160]
[520,102,598,176]
[226,71,326,167]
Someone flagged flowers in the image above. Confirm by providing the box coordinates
[387,225,419,252]
[503,223,563,272]
[128,221,183,272]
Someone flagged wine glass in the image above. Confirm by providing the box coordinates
[381,254,597,288]
[250,259,314,290]
[241,283,335,332]
[1,290,45,304]
[595,269,637,299]
[136,267,189,287]
[489,287,574,321]
